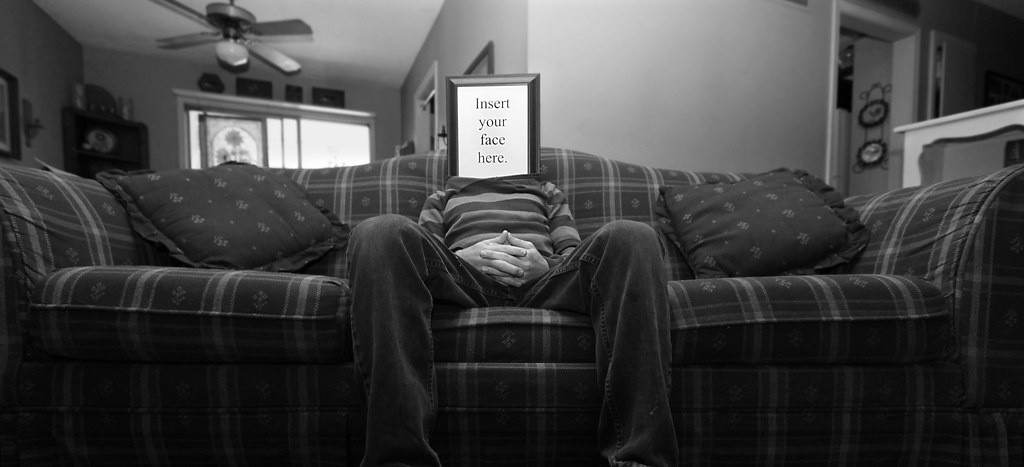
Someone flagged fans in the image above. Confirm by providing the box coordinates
[151,0,313,72]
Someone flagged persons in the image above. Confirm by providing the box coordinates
[347,176,680,467]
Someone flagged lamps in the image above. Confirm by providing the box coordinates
[206,0,257,67]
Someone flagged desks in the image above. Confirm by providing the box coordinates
[891,98,1024,189]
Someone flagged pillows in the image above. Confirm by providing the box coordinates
[93,160,350,273]
[653,166,869,280]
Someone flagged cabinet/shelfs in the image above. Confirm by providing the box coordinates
[62,106,150,181]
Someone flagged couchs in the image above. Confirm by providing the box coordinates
[0,147,1023,467]
